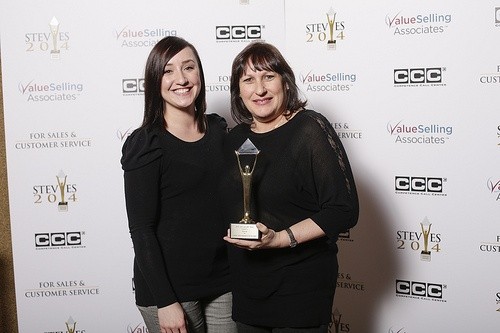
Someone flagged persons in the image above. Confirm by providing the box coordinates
[121,36,232,333]
[218,39,359,333]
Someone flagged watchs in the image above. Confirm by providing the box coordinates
[285,228,298,248]
